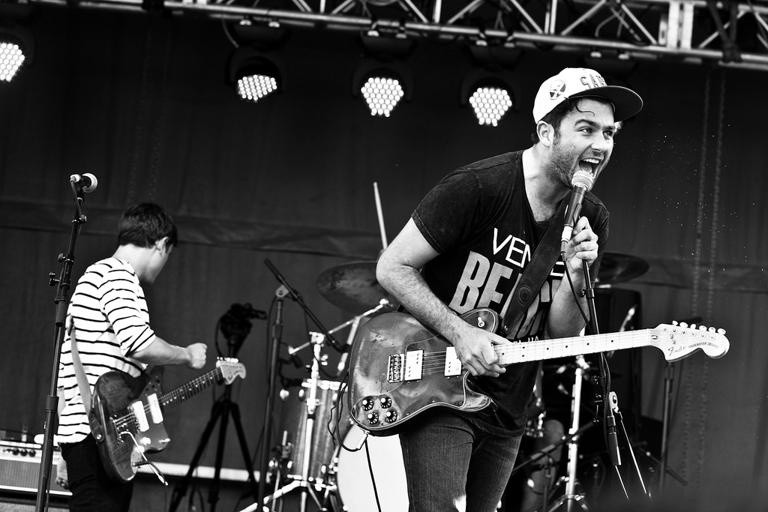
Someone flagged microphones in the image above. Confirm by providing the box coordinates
[70,172,99,195]
[337,316,361,372]
[557,169,596,260]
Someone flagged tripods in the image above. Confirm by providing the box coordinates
[239,303,381,512]
[169,340,258,512]
[543,353,600,512]
[504,288,687,512]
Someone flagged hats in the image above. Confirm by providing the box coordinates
[532,68,644,124]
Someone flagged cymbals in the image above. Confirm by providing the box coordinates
[594,251,650,285]
[315,261,412,317]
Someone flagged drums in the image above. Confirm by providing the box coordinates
[324,420,409,512]
[269,378,353,491]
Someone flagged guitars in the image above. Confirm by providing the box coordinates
[88,356,246,482]
[346,307,731,437]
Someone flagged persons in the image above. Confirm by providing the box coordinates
[57,202,209,512]
[375,68,643,512]
